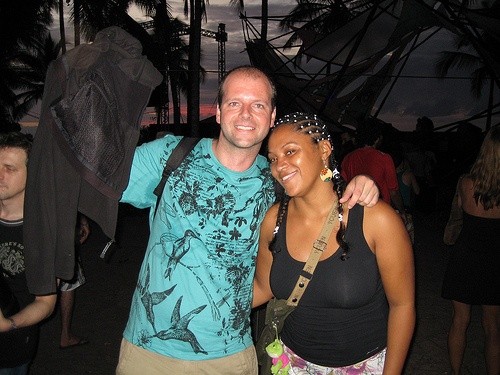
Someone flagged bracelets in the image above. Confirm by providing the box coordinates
[9,318,16,331]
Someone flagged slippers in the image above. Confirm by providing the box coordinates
[59,335,90,349]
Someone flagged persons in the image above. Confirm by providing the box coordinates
[117,65,381,375]
[250,112,415,375]
[328,114,499,375]
[0,132,90,375]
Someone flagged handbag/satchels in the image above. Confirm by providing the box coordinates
[255,299,295,375]
[443,174,470,245]
